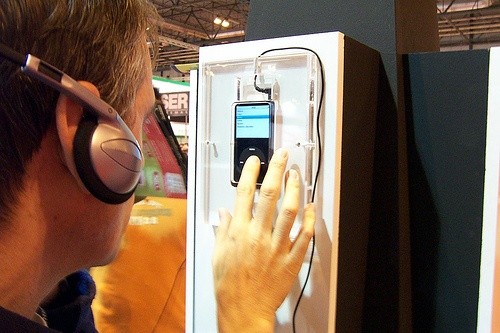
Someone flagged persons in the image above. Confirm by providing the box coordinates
[0,0,317,333]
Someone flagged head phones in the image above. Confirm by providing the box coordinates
[0,43,145,204]
